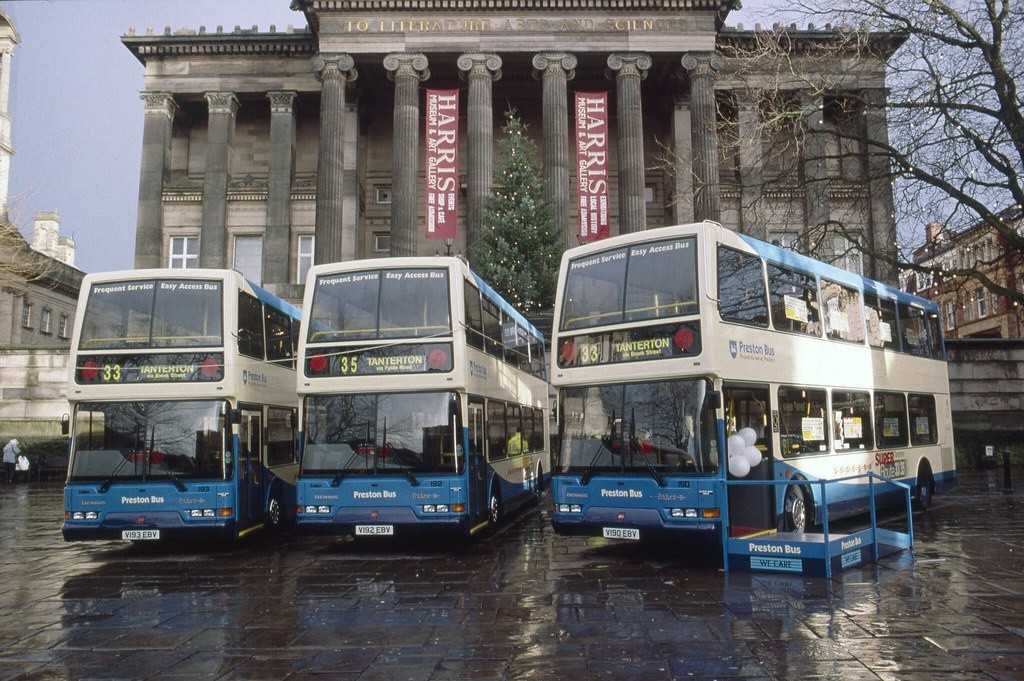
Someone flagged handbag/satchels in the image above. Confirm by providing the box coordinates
[14,454,29,471]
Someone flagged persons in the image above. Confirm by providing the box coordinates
[3,438,21,482]
[18,453,29,481]
[507,427,532,480]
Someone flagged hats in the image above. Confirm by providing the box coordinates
[9,438,19,445]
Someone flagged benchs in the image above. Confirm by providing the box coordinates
[0,454,72,484]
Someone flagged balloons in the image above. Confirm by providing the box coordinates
[727,427,762,477]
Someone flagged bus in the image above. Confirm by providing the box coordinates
[548,219,956,536]
[289,254,559,543]
[60,267,355,540]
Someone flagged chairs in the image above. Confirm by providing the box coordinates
[841,408,934,448]
